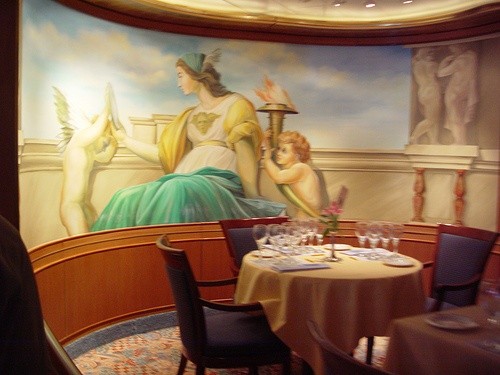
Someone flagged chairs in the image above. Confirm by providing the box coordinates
[156,216,500,375]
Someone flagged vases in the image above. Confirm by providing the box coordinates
[326,231,340,262]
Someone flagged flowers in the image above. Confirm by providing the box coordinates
[318,201,344,239]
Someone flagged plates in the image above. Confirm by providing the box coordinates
[324,243,351,250]
[424,313,481,329]
[252,250,281,257]
[383,257,413,267]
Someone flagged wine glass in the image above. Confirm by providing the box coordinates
[475,278,499,351]
[354,220,404,261]
[252,217,324,264]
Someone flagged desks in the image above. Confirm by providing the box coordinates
[381,305,500,375]
[233,243,425,375]
